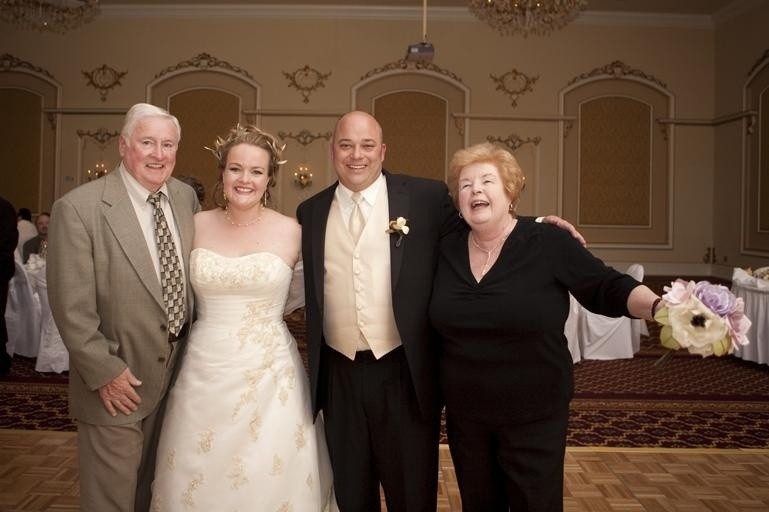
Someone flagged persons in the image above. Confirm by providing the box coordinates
[0,196,50,381]
[295,111,587,512]
[46,103,203,512]
[429,142,668,512]
[150,123,340,512]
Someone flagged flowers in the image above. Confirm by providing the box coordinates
[650,275,753,371]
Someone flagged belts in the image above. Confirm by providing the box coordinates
[168,324,190,342]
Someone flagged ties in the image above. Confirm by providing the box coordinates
[147,193,186,338]
[348,194,366,243]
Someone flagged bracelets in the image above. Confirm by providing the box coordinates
[651,298,663,319]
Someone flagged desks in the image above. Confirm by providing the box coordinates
[727,268,769,365]
